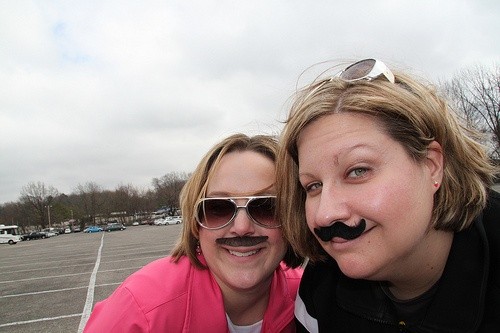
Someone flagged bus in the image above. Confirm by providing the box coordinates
[0,224,25,245]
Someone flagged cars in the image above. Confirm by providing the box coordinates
[131,210,183,226]
[20,222,126,241]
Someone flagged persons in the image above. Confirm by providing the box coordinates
[275,59,500,333]
[82,133,305,333]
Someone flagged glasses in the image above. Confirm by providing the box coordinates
[302,58,395,103]
[195,196,285,229]
